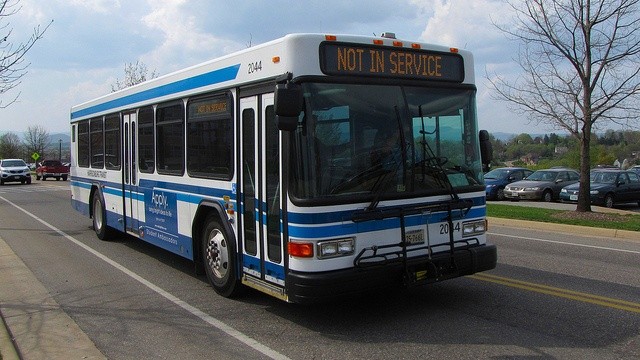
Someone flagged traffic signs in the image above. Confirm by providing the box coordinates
[32,153,40,160]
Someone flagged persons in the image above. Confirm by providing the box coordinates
[302,113,349,181]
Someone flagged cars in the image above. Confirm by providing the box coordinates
[559,169,640,208]
[35,159,70,181]
[483,168,534,201]
[502,168,581,202]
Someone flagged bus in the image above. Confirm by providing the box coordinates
[69,32,497,305]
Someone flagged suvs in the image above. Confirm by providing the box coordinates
[0,159,31,184]
